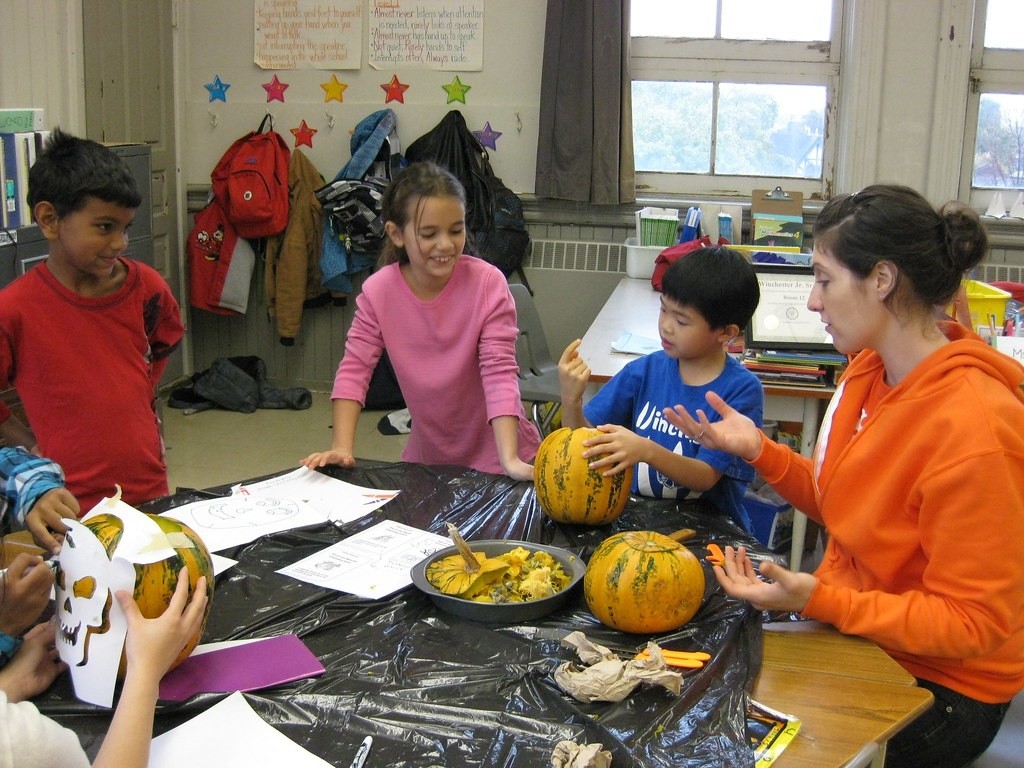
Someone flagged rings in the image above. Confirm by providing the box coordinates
[694,431,703,438]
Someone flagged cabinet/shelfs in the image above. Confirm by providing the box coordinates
[0,143,154,289]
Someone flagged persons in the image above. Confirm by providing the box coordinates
[1,124,186,518]
[558,247,764,533]
[299,160,544,481]
[663,184,1024,768]
[0,565,210,768]
[0,446,80,670]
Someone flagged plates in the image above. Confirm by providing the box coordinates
[410,539,586,620]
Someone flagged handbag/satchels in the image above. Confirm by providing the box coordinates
[650,236,728,292]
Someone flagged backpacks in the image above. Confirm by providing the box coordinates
[209,113,290,238]
[456,110,530,279]
[314,130,397,251]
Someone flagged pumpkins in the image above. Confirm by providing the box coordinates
[585,532,705,634]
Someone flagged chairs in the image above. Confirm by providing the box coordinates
[509,284,562,441]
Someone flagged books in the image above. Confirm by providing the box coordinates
[735,348,847,388]
[0,131,49,229]
[634,208,680,247]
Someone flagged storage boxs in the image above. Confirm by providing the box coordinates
[945,279,1012,335]
[738,498,793,554]
[749,247,814,267]
[624,236,681,279]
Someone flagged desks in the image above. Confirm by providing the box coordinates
[747,624,935,767]
[576,274,835,574]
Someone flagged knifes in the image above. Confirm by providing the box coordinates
[614,652,703,668]
[606,645,711,661]
